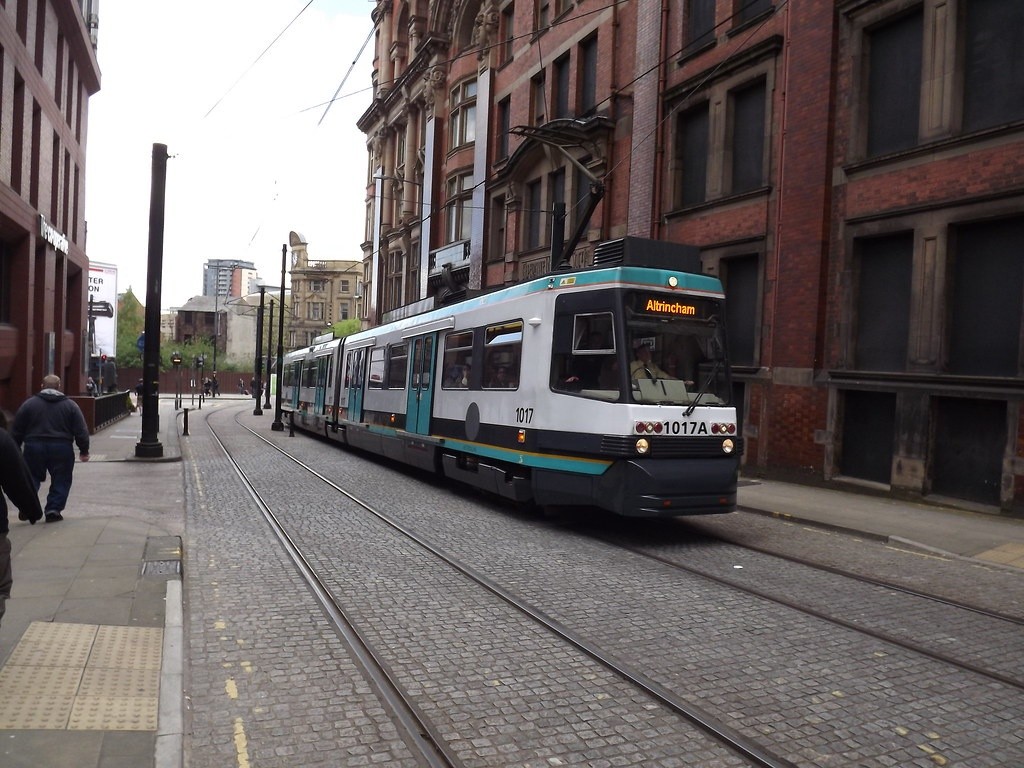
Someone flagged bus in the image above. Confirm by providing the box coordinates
[281,115,742,525]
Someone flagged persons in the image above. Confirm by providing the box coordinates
[11,375,90,521]
[238,378,245,394]
[213,378,220,397]
[135,379,143,416]
[662,334,705,392]
[0,410,43,623]
[443,364,471,388]
[87,377,99,396]
[488,366,516,388]
[107,383,118,392]
[203,376,212,396]
[630,343,694,391]
[250,377,255,395]
[551,332,617,390]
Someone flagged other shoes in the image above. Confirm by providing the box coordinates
[19,512,29,522]
[46,514,62,523]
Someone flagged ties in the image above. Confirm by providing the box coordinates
[645,364,651,378]
